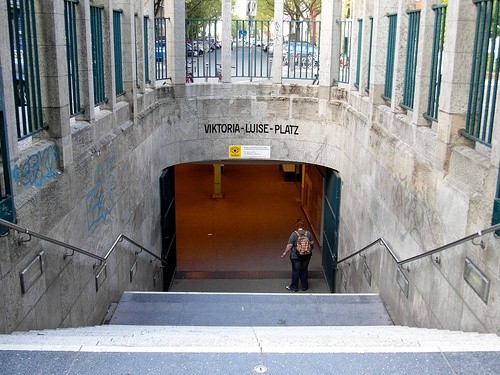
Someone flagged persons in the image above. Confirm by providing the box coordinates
[281,217,314,291]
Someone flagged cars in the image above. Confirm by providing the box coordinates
[155,37,219,60]
[255,39,315,67]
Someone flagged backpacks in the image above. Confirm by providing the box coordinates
[295,230,311,255]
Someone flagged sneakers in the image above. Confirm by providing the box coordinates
[286,285,290,290]
